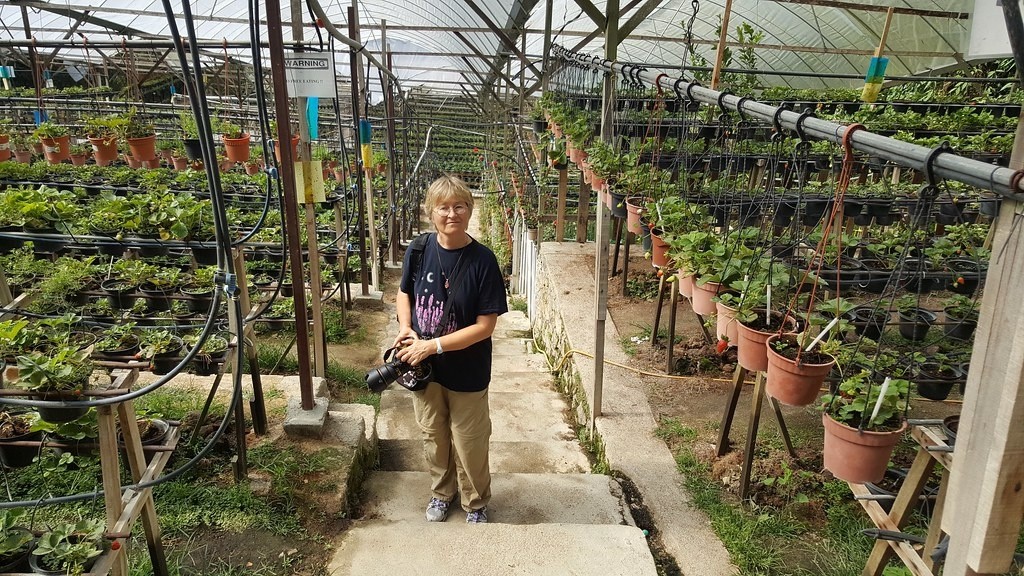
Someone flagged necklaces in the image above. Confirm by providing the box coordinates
[436,235,466,288]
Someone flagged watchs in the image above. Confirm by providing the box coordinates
[435,337,442,354]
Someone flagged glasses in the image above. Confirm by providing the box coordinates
[429,203,471,216]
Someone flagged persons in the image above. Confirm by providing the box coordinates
[396,174,507,522]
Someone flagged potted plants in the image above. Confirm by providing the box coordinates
[493,20,1022,482]
[392,95,492,251]
[0,78,388,576]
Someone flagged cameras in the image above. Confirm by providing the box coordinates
[364,341,434,393]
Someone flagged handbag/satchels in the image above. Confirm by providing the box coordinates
[389,335,434,391]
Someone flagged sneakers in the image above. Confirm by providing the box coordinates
[426,496,488,523]
